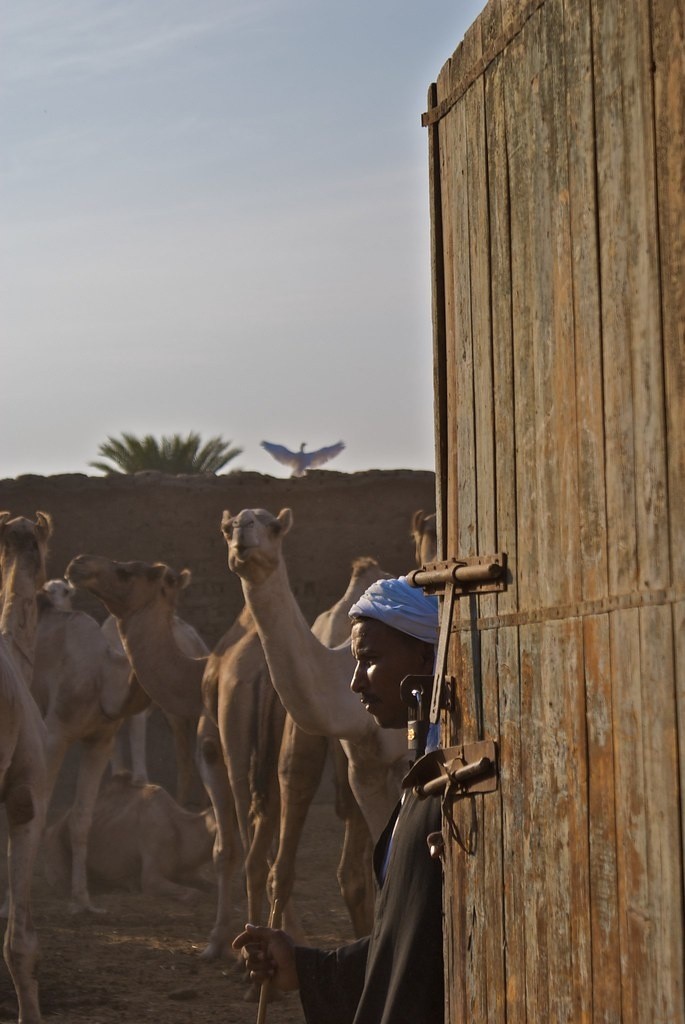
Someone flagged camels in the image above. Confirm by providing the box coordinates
[0,510,227,1024]
[201,507,472,1023]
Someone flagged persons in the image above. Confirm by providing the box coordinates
[229,577,448,1023]
